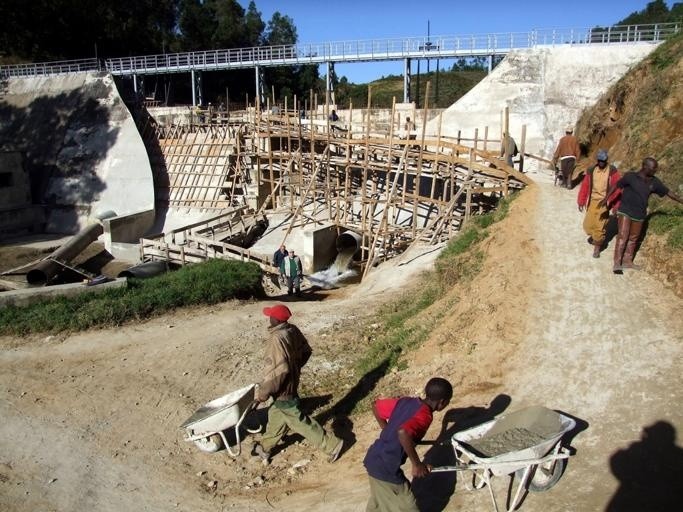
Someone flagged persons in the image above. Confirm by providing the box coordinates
[195,103,204,125]
[273,243,288,285]
[270,103,280,125]
[298,106,310,132]
[552,127,579,190]
[500,130,518,169]
[327,108,339,135]
[217,102,228,124]
[361,377,453,511]
[249,305,345,464]
[279,250,304,298]
[206,102,214,124]
[595,156,682,273]
[401,117,420,153]
[577,149,622,259]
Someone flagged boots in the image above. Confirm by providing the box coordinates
[588,236,602,258]
[562,174,572,189]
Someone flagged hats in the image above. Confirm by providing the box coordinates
[597,151,607,161]
[566,127,573,132]
[263,304,292,321]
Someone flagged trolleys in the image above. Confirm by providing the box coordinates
[488,154,515,175]
[399,135,421,151]
[330,124,350,139]
[176,383,267,458]
[550,161,577,188]
[411,404,579,512]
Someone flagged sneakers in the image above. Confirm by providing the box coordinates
[327,439,344,463]
[613,263,639,274]
[250,443,270,461]
